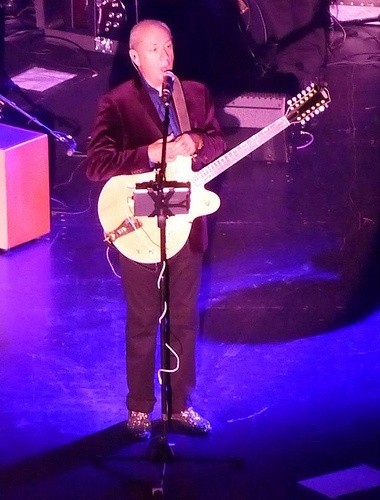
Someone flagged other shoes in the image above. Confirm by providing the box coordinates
[161,405,213,437]
[126,409,153,441]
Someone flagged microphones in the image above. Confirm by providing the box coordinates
[161,71,176,102]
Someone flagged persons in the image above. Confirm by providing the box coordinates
[85,19,227,439]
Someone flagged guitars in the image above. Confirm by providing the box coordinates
[94,76,332,265]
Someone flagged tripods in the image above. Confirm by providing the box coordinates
[82,99,245,500]
